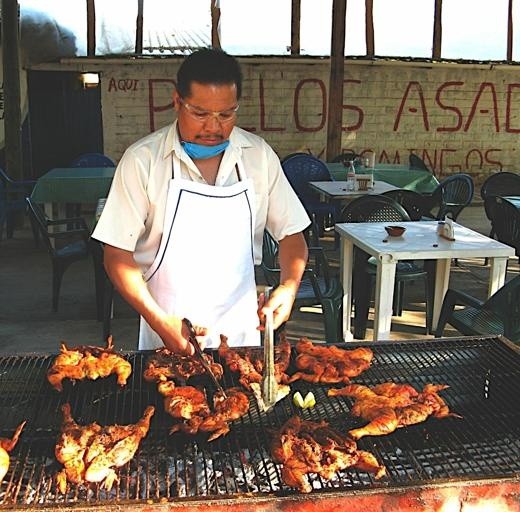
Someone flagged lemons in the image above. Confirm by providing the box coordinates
[293,392,316,412]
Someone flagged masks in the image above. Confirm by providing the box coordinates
[180,139,230,160]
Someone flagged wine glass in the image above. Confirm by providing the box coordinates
[346,160,355,193]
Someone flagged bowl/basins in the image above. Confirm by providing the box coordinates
[343,160,355,167]
[385,226,406,236]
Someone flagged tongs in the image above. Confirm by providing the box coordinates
[262,287,280,406]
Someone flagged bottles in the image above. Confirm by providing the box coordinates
[437,220,445,236]
[365,157,369,167]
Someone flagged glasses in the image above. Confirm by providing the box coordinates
[178,96,241,124]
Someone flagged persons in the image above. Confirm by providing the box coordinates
[89,44,311,357]
